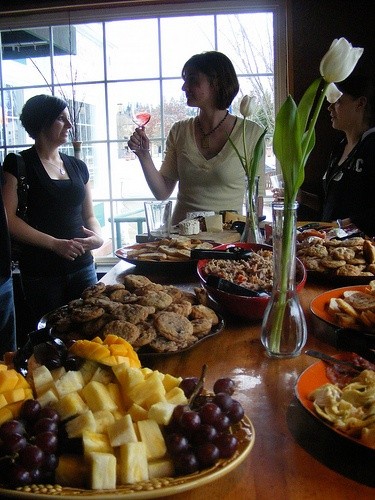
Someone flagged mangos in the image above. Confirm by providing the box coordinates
[69,335,142,366]
[0,364,34,424]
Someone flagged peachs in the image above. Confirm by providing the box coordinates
[33,366,187,490]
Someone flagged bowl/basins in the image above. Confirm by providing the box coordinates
[197,242,307,323]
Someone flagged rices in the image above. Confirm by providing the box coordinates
[204,248,273,289]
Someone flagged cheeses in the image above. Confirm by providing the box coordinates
[225,212,246,224]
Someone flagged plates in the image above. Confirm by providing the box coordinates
[0,409,254,500]
[310,285,375,336]
[36,308,224,357]
[295,349,375,448]
[304,276,375,286]
[112,240,225,270]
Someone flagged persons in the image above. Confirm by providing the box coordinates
[1,95,103,329]
[0,165,16,361]
[127,51,265,228]
[272,58,375,236]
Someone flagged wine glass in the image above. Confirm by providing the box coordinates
[131,101,152,151]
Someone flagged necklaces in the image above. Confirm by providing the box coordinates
[43,158,66,175]
[198,110,229,149]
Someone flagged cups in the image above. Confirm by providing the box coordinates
[270,174,284,201]
[144,200,172,239]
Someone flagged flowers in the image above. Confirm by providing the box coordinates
[227,95,271,241]
[270,36,364,354]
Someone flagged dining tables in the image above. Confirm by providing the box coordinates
[0,220,375,500]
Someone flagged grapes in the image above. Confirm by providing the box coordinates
[163,365,244,472]
[0,399,61,487]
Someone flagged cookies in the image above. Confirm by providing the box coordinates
[51,274,218,351]
[296,236,375,276]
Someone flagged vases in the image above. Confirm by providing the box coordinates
[239,176,265,245]
[261,201,309,358]
[72,140,83,162]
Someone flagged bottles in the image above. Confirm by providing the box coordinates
[220,210,238,222]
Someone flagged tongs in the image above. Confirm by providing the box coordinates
[207,275,270,297]
[190,247,254,261]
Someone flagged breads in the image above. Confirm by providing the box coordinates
[328,280,375,331]
[126,234,214,261]
